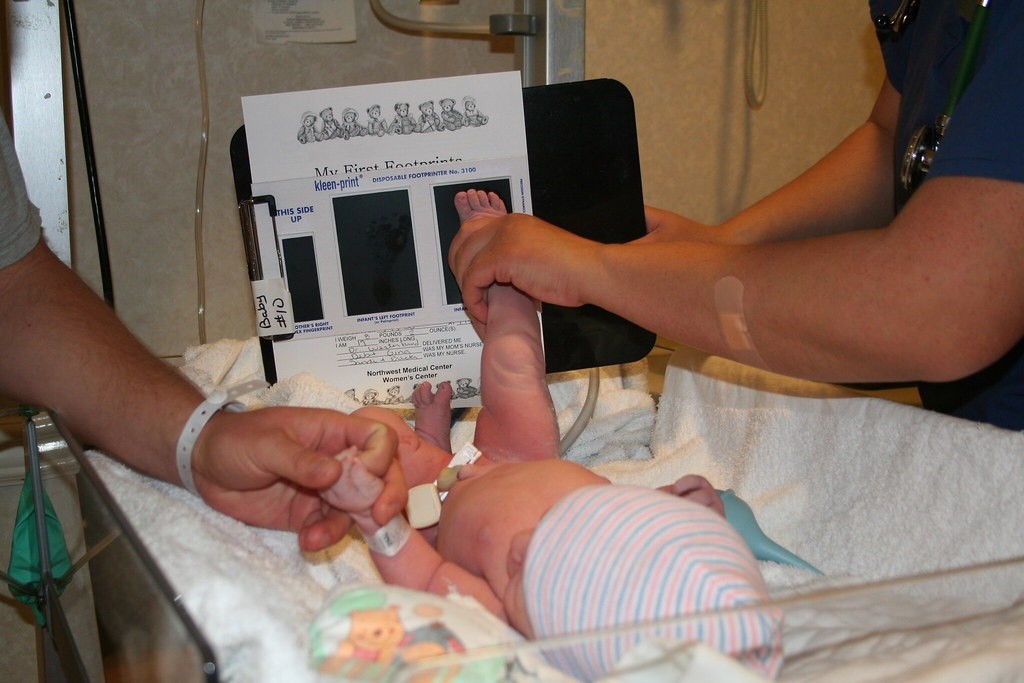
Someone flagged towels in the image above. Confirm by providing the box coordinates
[86,347,1024,683]
[177,335,264,411]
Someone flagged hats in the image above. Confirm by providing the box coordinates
[523,485,783,683]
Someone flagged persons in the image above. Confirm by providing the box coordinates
[0,107,409,552]
[448,0,1024,431]
[348,189,784,683]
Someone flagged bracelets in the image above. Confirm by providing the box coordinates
[176,379,270,497]
[353,513,411,557]
